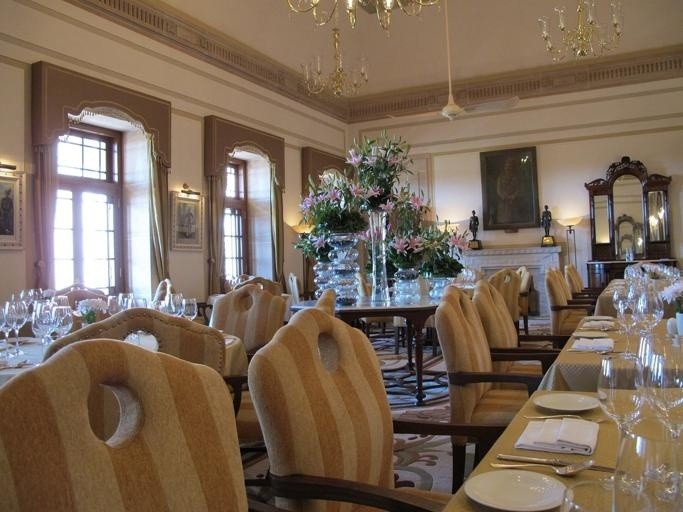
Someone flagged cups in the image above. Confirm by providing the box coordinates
[561,480,653,511]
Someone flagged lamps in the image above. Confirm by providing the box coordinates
[556,217,583,271]
[284,0,444,41]
[536,1,628,69]
[296,1,370,104]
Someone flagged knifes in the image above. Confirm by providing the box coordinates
[566,348,623,353]
[495,452,626,474]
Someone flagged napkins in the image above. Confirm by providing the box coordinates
[566,337,616,354]
[514,417,599,455]
[583,320,616,331]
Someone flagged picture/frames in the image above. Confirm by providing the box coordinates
[479,145,542,233]
[167,188,205,253]
[0,158,26,251]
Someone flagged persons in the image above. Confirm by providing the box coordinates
[185,206,197,236]
[468,209,478,241]
[0,187,12,235]
[540,205,551,237]
[495,156,523,224]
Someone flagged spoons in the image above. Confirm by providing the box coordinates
[522,414,605,423]
[578,326,611,331]
[488,458,594,477]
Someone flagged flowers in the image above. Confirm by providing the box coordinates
[285,125,480,315]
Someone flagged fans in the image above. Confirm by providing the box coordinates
[375,1,522,129]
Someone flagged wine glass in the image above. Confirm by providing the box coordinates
[0,280,198,367]
[594,263,683,512]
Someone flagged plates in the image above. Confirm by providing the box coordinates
[583,316,612,321]
[571,330,607,338]
[463,468,574,511]
[533,393,599,412]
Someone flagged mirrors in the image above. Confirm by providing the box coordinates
[583,154,672,261]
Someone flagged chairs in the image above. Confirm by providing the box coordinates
[485,268,520,347]
[545,264,605,350]
[435,284,560,495]
[1,274,292,487]
[1,338,249,512]
[249,308,455,512]
[354,269,484,357]
[515,265,531,336]
[472,278,572,375]
